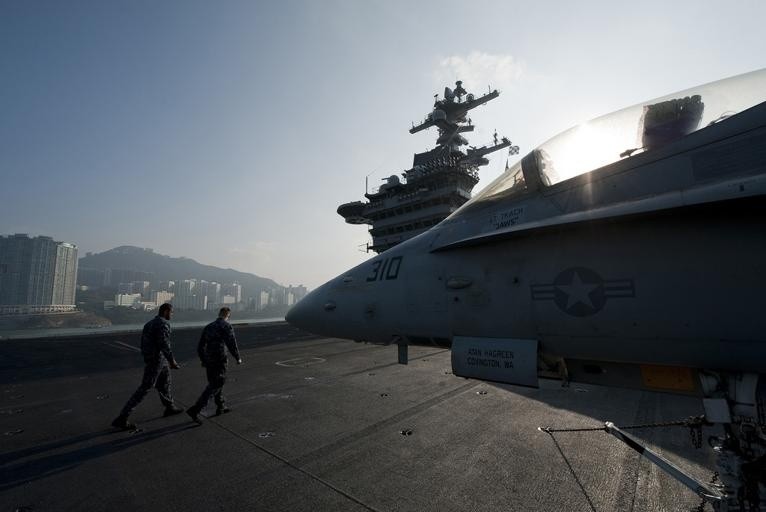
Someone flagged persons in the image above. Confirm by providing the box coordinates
[186,306,244,426]
[110,303,185,431]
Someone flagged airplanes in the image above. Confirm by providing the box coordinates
[282,66,766,407]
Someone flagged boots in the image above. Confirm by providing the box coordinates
[164,403,184,415]
[112,417,136,430]
[185,406,203,425]
[215,399,232,415]
